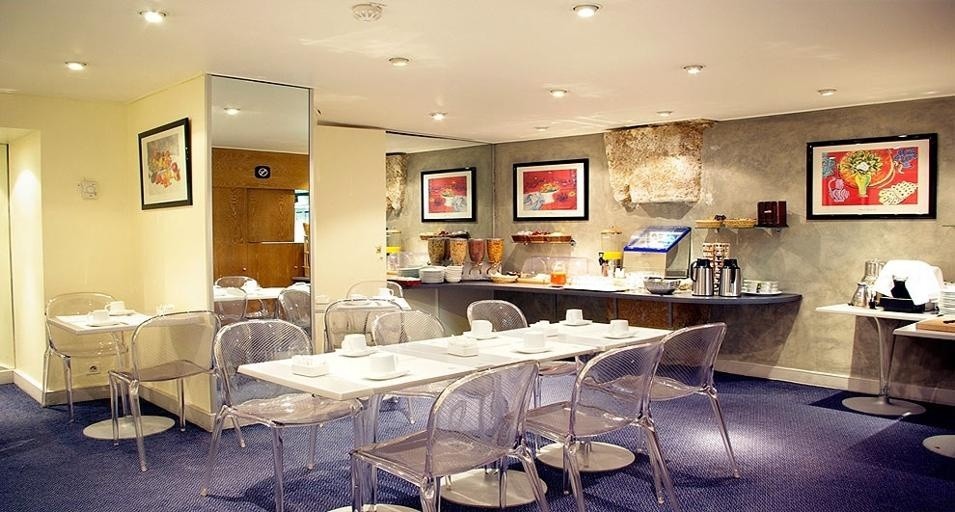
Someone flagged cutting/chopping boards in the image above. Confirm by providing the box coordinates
[916,315,955,333]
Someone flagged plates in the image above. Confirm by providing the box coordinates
[362,371,411,381]
[558,319,592,325]
[335,348,377,357]
[605,331,635,338]
[418,265,464,284]
[447,350,482,357]
[934,281,955,317]
[84,309,138,326]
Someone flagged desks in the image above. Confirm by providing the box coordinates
[445,273,799,333]
[815,300,937,415]
[889,321,953,463]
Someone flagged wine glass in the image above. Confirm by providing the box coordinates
[427,239,505,277]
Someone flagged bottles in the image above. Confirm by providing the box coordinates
[551,261,568,285]
[690,259,741,297]
[850,258,888,307]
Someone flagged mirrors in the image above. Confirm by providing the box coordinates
[386,130,497,289]
[202,73,312,344]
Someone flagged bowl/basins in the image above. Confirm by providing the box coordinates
[645,278,681,295]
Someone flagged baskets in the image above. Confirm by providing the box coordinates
[511,235,571,242]
[695,220,720,227]
[725,220,756,227]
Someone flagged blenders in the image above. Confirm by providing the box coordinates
[600,226,624,278]
[386,229,403,274]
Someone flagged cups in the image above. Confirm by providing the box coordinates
[341,335,368,352]
[744,279,781,294]
[369,357,397,374]
[454,335,479,353]
[566,307,584,321]
[91,300,125,319]
[467,318,561,354]
[610,318,628,333]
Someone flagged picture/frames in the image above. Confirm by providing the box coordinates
[511,158,591,222]
[419,166,478,224]
[138,116,193,212]
[803,132,936,220]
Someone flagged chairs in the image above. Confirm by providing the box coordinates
[44,293,245,473]
[346,282,404,299]
[201,298,740,510]
[214,276,312,335]
